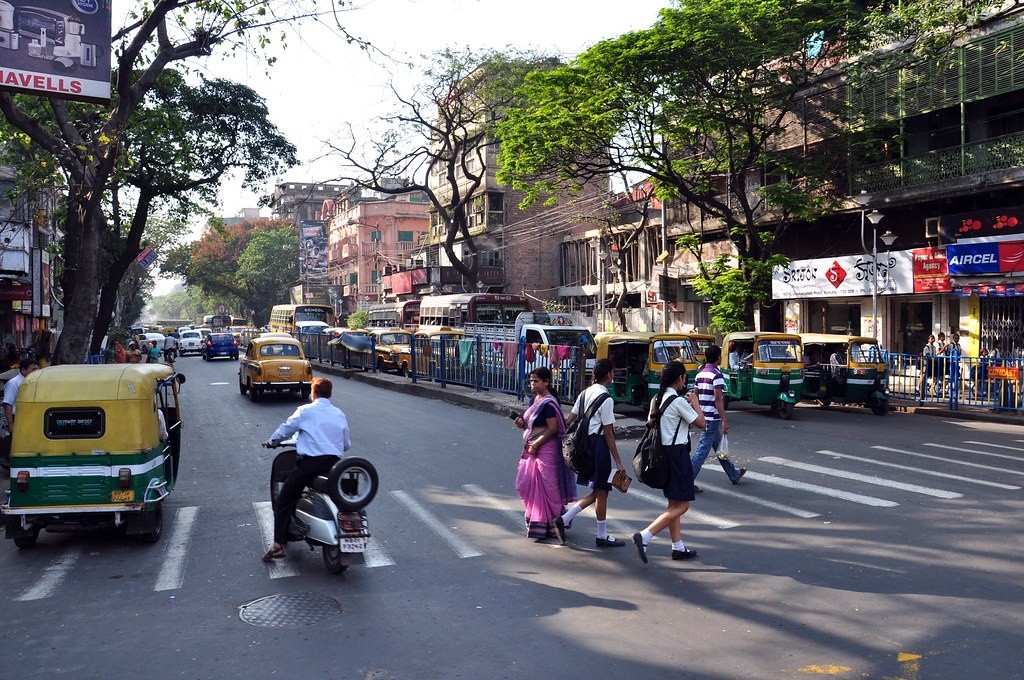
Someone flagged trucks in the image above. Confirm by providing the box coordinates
[462,311,597,395]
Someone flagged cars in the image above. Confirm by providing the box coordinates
[131,325,239,361]
[239,337,313,401]
[296,320,464,378]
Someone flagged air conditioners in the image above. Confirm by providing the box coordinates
[925,217,939,238]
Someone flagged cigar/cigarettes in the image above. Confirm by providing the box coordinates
[684,385,686,387]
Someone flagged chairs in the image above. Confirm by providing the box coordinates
[278,345,294,355]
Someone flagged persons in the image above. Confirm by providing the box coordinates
[514,367,577,543]
[260,377,351,561]
[830,345,847,383]
[164,330,180,362]
[106,331,142,363]
[147,340,162,363]
[979,340,1006,396]
[553,359,626,547]
[631,361,706,563]
[729,343,749,372]
[918,332,961,398]
[2,359,35,435]
[689,345,747,493]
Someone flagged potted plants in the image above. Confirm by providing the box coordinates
[472,196,485,211]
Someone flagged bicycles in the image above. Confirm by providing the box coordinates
[927,376,1002,398]
[162,347,176,364]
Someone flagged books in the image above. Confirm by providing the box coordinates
[607,469,632,493]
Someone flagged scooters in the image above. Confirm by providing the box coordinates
[262,430,379,574]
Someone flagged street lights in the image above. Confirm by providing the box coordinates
[283,240,312,304]
[588,238,617,331]
[329,288,343,328]
[347,218,380,305]
[858,188,897,338]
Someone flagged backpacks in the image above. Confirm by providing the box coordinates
[561,388,613,476]
[915,345,930,370]
[631,391,685,489]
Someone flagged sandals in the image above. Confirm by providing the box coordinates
[694,485,704,493]
[732,467,747,485]
[262,546,286,561]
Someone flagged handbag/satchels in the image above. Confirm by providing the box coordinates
[146,357,150,363]
[716,434,731,461]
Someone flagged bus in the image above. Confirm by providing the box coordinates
[418,292,534,327]
[269,304,334,334]
[367,300,421,330]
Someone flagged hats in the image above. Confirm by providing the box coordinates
[128,340,135,348]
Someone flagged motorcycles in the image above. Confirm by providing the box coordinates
[721,331,804,420]
[795,334,890,415]
[0,362,186,547]
[594,331,730,419]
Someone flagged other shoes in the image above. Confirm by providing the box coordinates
[595,534,625,548]
[671,547,698,560]
[553,516,566,545]
[631,532,648,564]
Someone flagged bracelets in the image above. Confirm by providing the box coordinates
[528,444,538,449]
